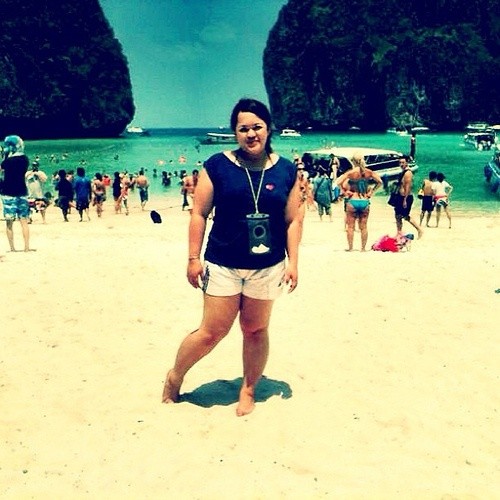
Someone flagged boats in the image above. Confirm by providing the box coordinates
[127,126,146,133]
[280,127,301,139]
[484,147,500,202]
[197,132,239,144]
[301,147,418,184]
[464,123,500,151]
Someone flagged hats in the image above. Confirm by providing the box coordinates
[293,153,300,160]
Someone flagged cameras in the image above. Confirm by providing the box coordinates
[246,213,272,254]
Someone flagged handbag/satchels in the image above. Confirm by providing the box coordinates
[313,192,317,201]
[387,191,401,207]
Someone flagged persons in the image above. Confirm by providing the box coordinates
[162,99,304,417]
[0,135,500,251]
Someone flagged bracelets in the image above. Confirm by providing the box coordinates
[189,254,199,260]
